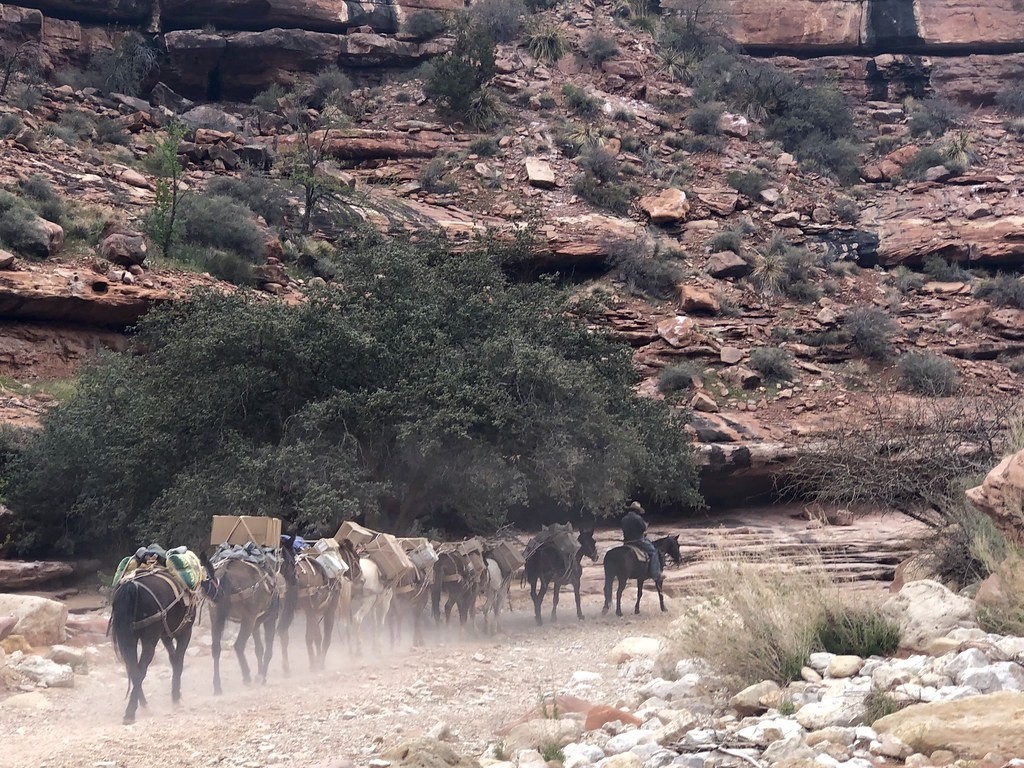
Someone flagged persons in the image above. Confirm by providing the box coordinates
[621,502,667,582]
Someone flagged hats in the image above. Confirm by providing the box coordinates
[626,501,645,514]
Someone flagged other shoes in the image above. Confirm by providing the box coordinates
[658,575,666,582]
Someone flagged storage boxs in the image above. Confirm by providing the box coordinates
[306,520,526,580]
[210,515,282,549]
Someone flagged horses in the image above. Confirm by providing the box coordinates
[468,556,511,634]
[108,550,226,726]
[601,533,682,617]
[290,537,433,672]
[205,531,298,697]
[430,551,490,629]
[525,528,600,627]
